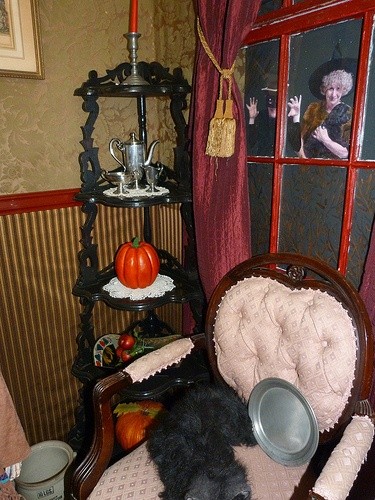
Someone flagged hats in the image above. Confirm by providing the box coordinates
[309,46,358,100]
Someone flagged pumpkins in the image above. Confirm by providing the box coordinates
[113,401,163,450]
[114,237,159,290]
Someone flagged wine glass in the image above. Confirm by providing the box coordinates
[104,172,133,194]
[146,168,160,192]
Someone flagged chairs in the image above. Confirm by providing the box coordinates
[65,250,375,500]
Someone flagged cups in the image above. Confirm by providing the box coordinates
[14,440,75,500]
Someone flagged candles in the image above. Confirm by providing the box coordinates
[128,0,138,33]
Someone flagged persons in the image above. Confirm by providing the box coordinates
[246,74,302,156]
[299,45,359,160]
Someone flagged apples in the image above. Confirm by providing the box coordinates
[116,334,135,360]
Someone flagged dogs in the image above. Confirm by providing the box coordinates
[144,379,258,500]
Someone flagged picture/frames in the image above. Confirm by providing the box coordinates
[0,0,45,81]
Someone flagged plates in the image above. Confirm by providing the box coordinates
[247,377,320,468]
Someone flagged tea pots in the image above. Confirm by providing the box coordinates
[109,132,160,185]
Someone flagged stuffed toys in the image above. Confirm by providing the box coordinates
[148,384,258,500]
